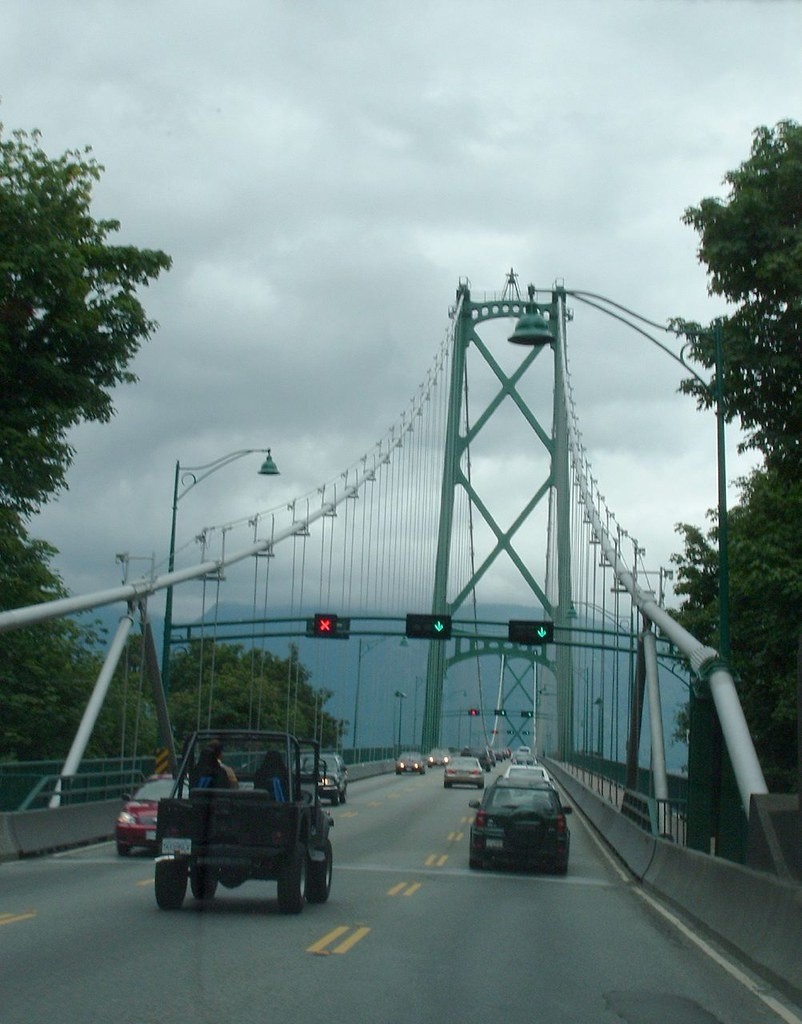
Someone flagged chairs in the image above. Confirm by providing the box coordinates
[495,791,512,805]
[253,750,286,794]
[189,746,231,790]
[532,793,547,807]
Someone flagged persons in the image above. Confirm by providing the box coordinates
[206,740,240,789]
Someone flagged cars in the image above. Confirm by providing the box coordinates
[506,766,555,795]
[461,747,511,772]
[394,751,426,774]
[427,749,452,767]
[116,774,190,856]
[444,756,484,789]
[511,746,537,766]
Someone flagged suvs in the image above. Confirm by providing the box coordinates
[469,776,573,876]
[153,729,332,913]
[292,752,348,804]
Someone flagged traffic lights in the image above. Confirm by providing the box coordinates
[522,731,529,735]
[406,613,452,638]
[494,710,506,716]
[468,709,477,716]
[314,613,337,635]
[521,711,532,717]
[508,620,553,643]
[507,731,514,734]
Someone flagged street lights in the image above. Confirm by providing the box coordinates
[155,449,283,751]
[505,284,735,855]
[548,661,589,755]
[566,599,634,788]
[353,635,410,746]
[440,689,469,749]
